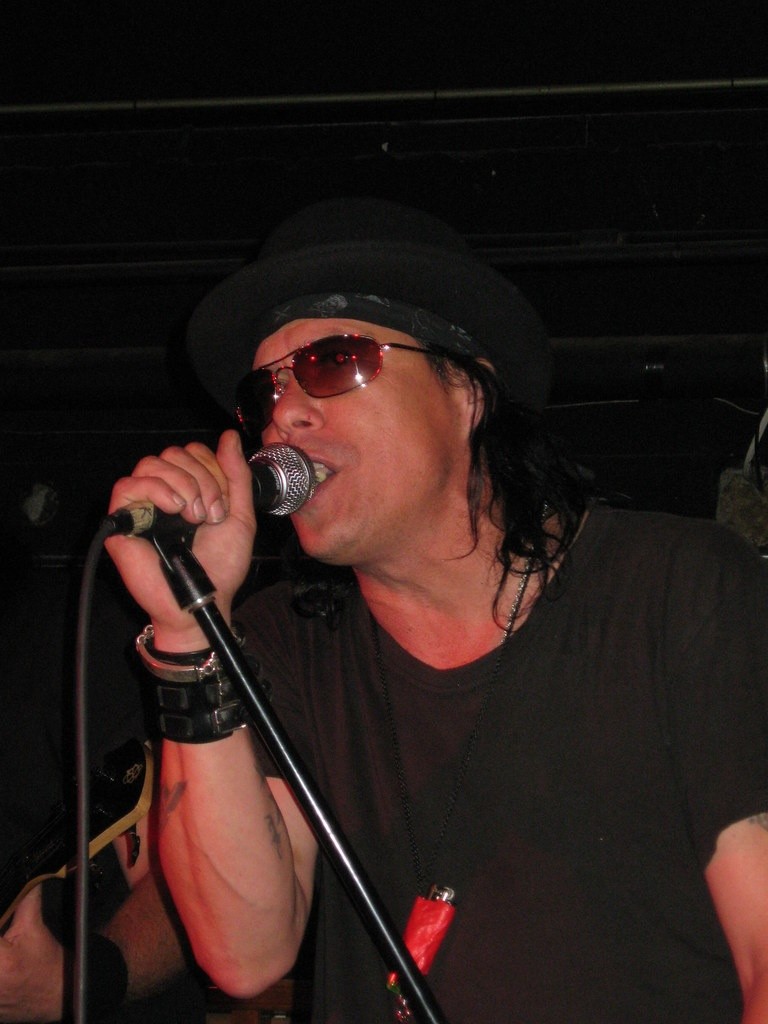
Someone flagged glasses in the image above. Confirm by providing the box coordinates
[234,335,445,434]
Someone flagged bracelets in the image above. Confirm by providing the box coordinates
[131,624,248,745]
[55,924,129,1024]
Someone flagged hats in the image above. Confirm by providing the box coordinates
[186,197,550,419]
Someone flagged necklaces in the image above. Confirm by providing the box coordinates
[366,500,548,1024]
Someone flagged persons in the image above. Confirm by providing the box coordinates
[0,528,206,1024]
[102,196,766,1023]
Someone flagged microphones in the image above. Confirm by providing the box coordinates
[108,442,316,536]
